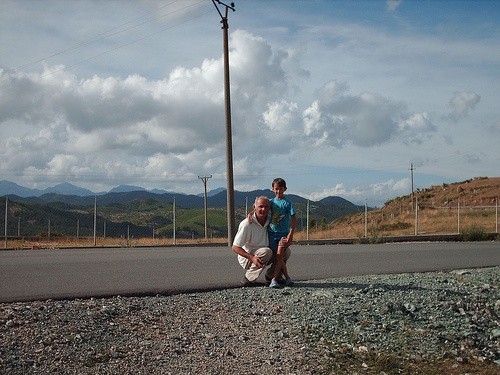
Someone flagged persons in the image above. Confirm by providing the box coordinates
[248,178,296,288]
[231,196,291,285]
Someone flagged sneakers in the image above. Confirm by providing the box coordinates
[265,274,286,284]
[269,281,280,288]
[240,277,250,287]
[285,277,295,286]
